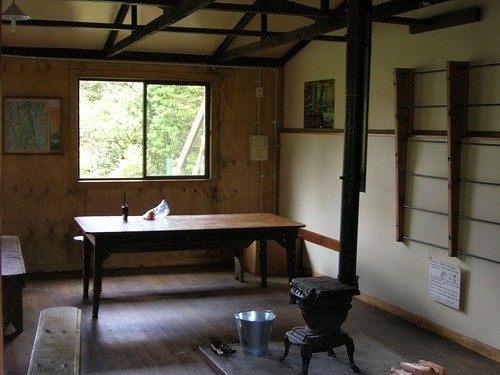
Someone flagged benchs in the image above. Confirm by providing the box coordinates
[26,306,83,375]
[0,235,28,336]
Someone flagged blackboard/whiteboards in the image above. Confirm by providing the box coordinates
[428,258,462,310]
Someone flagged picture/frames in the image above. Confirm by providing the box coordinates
[2,95,65,158]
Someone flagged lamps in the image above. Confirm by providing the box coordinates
[0,0,32,32]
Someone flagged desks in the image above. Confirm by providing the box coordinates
[74,212,306,318]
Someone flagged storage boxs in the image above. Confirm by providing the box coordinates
[243,234,302,276]
